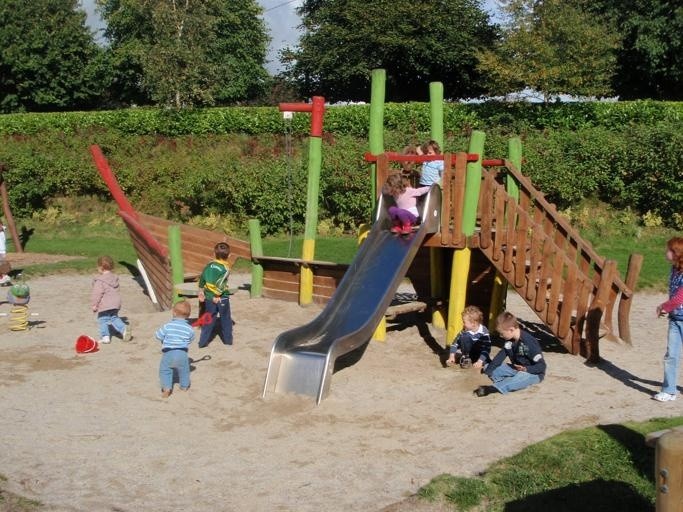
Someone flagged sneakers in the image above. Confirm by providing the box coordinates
[0,275,11,284]
[98,336,111,344]
[123,324,132,341]
[462,359,472,369]
[391,221,412,235]
[477,386,488,397]
[654,391,677,402]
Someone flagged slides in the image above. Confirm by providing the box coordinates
[264,182,442,407]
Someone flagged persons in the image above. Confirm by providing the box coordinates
[472,311,546,397]
[91,255,131,344]
[381,173,430,236]
[155,300,192,396]
[445,305,490,367]
[417,141,445,201]
[654,238,683,401]
[197,242,234,348]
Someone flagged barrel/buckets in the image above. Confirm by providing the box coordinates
[77,336,99,353]
